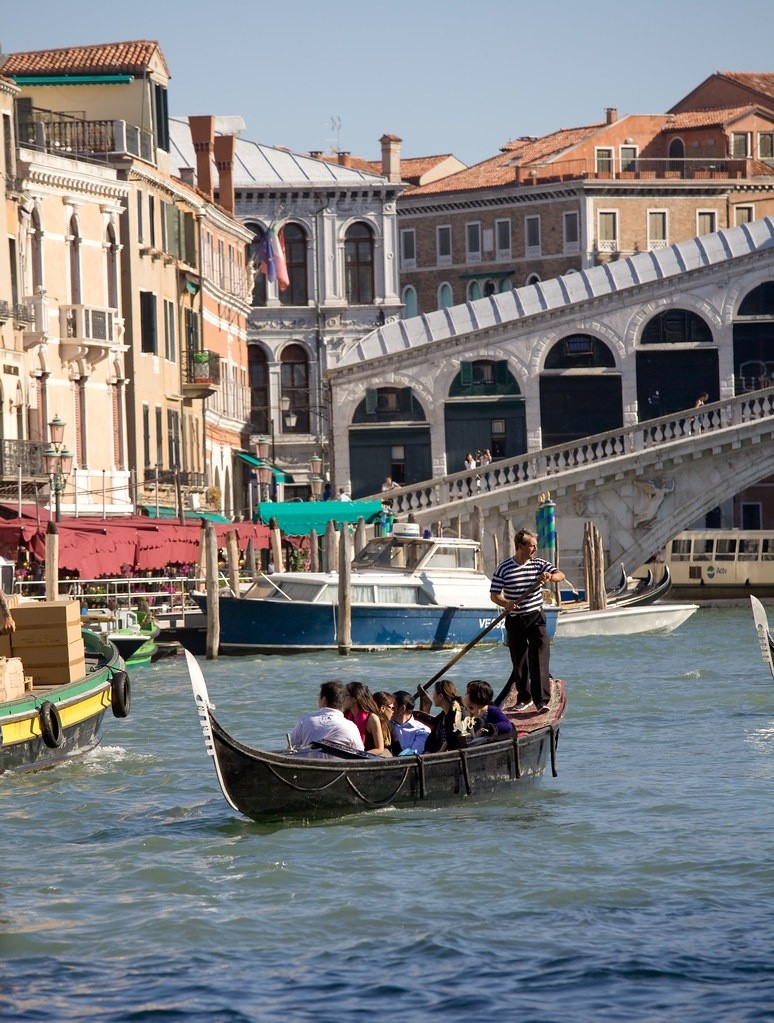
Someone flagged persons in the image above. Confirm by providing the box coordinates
[690,392,709,431]
[372,691,396,759]
[426,678,467,752]
[322,483,333,502]
[283,680,366,759]
[381,475,401,493]
[464,452,477,497]
[475,449,493,491]
[387,689,432,756]
[340,681,384,757]
[490,528,566,715]
[338,487,352,502]
[651,481,674,505]
[461,679,513,747]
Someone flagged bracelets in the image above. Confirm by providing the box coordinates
[550,574,553,580]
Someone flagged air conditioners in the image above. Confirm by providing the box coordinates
[376,393,398,411]
[473,364,492,384]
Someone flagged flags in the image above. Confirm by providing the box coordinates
[247,217,290,291]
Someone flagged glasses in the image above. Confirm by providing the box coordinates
[386,704,394,711]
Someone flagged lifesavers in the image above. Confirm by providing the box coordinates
[41,701,62,748]
[113,672,131,718]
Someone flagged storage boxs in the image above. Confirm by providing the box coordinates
[0,596,86,684]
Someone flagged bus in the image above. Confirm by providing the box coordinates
[653,527,774,587]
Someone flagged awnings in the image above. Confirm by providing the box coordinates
[182,282,198,296]
[233,448,295,484]
[254,499,390,549]
[0,503,271,584]
[6,75,137,86]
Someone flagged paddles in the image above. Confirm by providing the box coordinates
[413,576,549,700]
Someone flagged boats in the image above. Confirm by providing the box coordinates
[179,647,566,825]
[750,594,774,676]
[554,563,699,638]
[0,629,131,780]
[190,522,563,655]
[82,609,180,668]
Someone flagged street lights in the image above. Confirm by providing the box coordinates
[256,435,272,575]
[308,453,323,571]
[43,414,73,521]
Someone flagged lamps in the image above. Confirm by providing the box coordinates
[277,387,331,411]
[285,405,327,431]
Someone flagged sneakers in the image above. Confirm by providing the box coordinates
[510,700,534,712]
[536,704,550,714]
